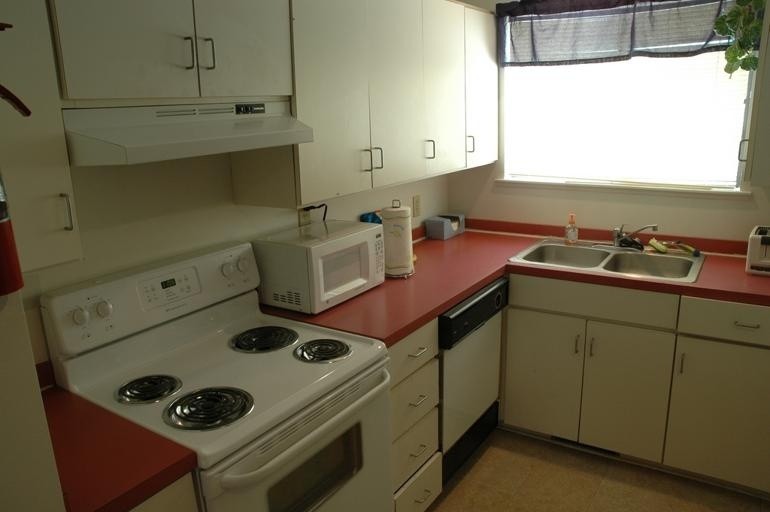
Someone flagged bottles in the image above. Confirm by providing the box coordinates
[380,200,414,279]
[564,212,579,244]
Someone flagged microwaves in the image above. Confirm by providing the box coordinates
[250,220,386,316]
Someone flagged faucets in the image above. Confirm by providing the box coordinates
[612,222,659,248]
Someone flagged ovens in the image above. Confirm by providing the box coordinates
[195,355,397,511]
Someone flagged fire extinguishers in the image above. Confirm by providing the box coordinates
[0,23,32,296]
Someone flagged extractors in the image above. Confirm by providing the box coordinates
[60,102,316,170]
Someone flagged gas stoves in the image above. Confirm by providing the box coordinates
[74,307,388,472]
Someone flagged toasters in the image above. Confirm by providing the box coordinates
[743,225,770,277]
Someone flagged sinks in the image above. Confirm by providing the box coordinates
[603,251,695,280]
[520,243,611,270]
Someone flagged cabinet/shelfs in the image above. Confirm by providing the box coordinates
[51,0,294,111]
[663,285,770,496]
[384,318,445,512]
[423,1,468,181]
[232,0,423,214]
[468,5,500,170]
[0,1,85,273]
[497,273,681,477]
